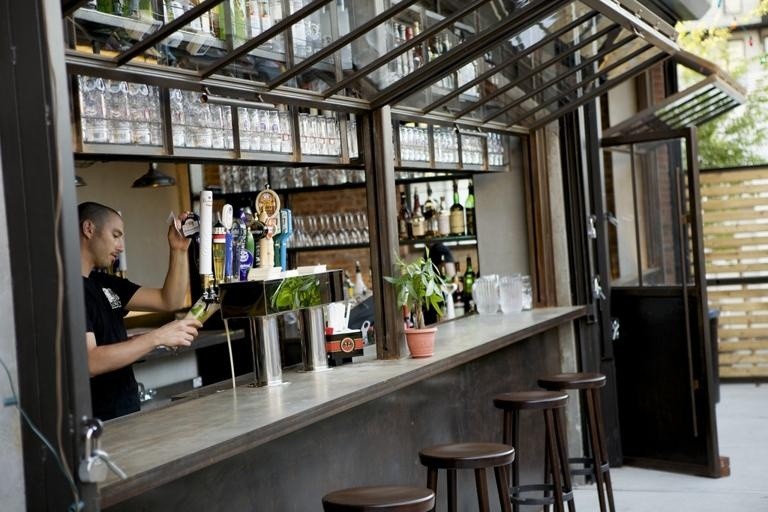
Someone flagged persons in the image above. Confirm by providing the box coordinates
[422,231,455,281]
[76,198,205,425]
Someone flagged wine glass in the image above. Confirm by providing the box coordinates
[286,212,371,247]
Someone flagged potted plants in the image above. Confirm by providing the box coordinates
[382,247,452,356]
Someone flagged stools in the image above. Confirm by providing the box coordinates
[535,374,615,512]
[419,441,515,512]
[322,486,436,512]
[492,390,576,512]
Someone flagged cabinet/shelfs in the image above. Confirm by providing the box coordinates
[187,163,480,386]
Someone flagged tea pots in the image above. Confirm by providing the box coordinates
[471,273,524,315]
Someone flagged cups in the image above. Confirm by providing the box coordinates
[237,105,294,153]
[76,74,164,146]
[218,164,410,194]
[169,87,234,149]
[398,125,507,166]
[156,296,221,355]
[521,276,533,311]
[298,113,360,158]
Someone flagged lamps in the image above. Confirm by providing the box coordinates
[131,159,176,189]
[75,175,87,186]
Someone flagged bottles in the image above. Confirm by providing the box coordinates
[392,20,498,95]
[397,181,477,242]
[438,255,475,317]
[401,301,418,329]
[63,1,357,68]
[342,260,372,300]
